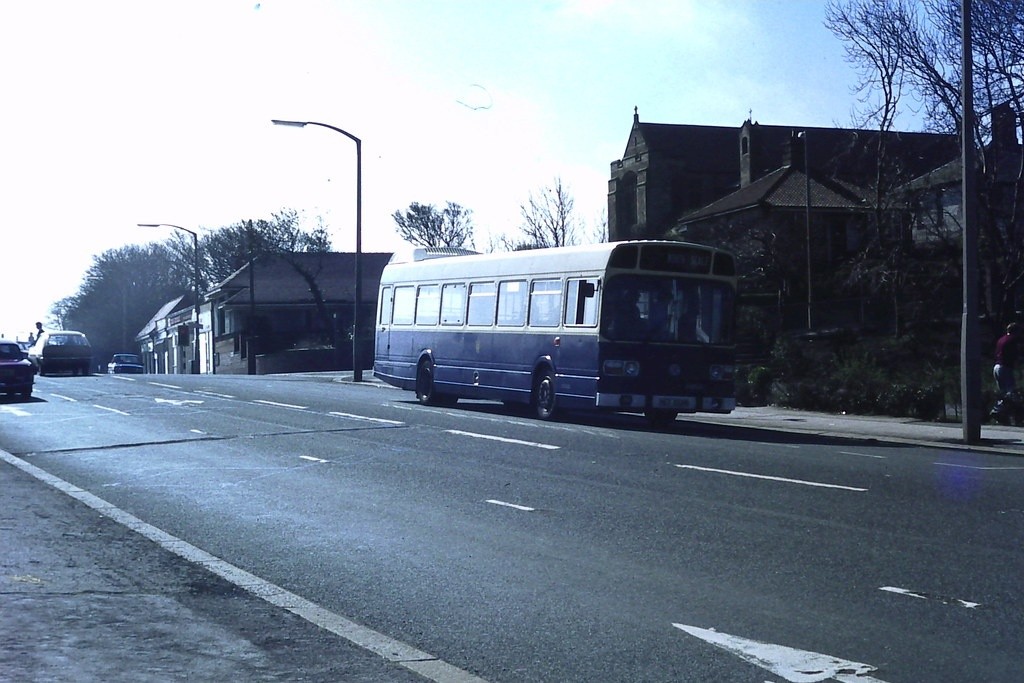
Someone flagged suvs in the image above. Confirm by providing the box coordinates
[31,330,93,377]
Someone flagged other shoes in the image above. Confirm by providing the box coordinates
[989,409,1005,422]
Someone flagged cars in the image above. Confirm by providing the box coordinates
[0,339,34,403]
[108,353,145,375]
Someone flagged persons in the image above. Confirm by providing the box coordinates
[990,323,1024,426]
[28,322,45,376]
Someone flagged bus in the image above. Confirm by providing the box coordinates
[372,239,742,430]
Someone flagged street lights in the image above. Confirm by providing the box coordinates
[87,274,129,354]
[138,224,200,374]
[270,119,364,383]
